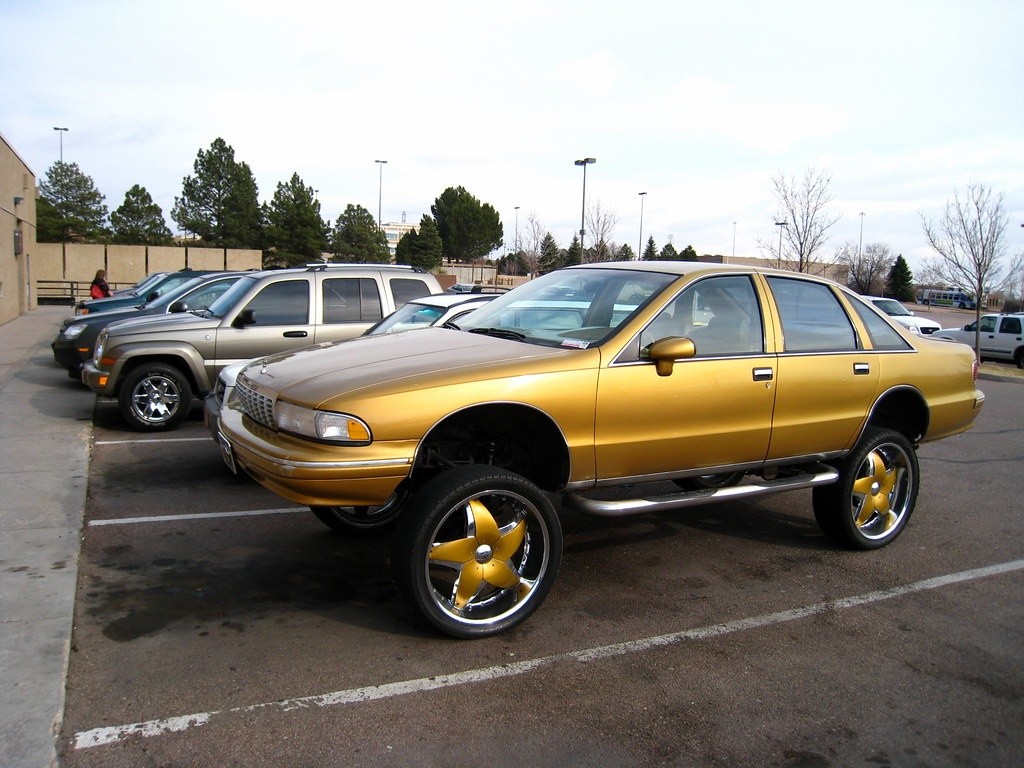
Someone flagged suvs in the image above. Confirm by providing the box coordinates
[82,262,446,430]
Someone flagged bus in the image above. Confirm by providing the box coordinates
[922,289,976,310]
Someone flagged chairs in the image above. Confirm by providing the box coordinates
[688,316,749,354]
[644,312,672,336]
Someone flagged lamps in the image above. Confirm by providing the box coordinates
[14,197,24,207]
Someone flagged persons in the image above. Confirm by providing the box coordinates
[90,270,113,299]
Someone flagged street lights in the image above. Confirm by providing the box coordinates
[774,222,788,271]
[575,158,595,267]
[514,206,520,267]
[638,191,645,257]
[374,160,387,233]
[54,127,70,279]
[860,212,866,256]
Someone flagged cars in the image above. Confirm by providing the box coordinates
[933,313,1024,370]
[855,293,942,336]
[915,297,923,304]
[216,260,987,640]
[51,269,355,380]
[203,284,642,437]
